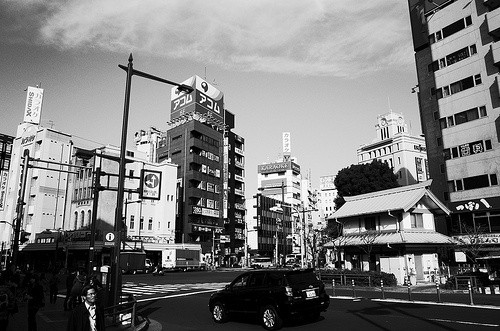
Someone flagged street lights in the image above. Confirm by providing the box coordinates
[107,52,195,315]
[274,203,304,268]
[0,221,20,287]
[258,181,287,268]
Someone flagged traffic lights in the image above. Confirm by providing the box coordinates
[20,230,32,244]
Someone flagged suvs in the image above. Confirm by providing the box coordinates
[208,266,331,331]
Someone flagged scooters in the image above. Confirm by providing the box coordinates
[64,271,103,312]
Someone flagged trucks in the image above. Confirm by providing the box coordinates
[119,251,154,276]
[161,245,210,273]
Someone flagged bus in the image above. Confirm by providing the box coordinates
[272,252,306,269]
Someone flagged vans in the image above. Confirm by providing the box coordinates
[249,257,274,270]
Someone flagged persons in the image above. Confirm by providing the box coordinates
[22,275,46,331]
[21,265,103,312]
[66,285,105,331]
